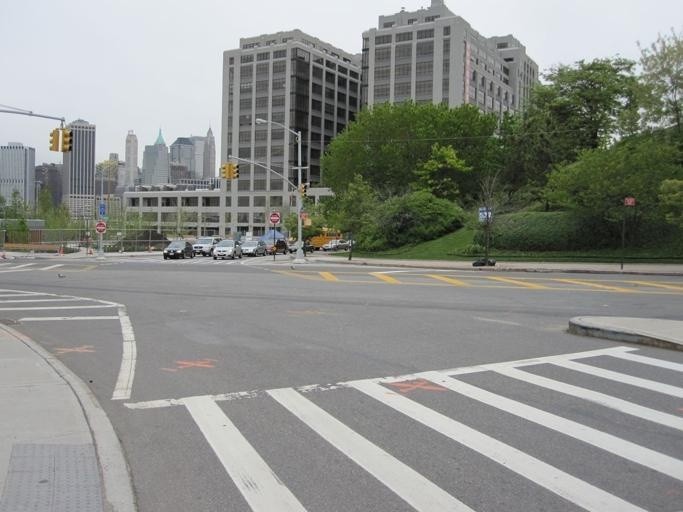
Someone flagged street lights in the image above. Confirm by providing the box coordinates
[254,117,307,265]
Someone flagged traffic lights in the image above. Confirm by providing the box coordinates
[300,183,307,198]
[230,163,240,180]
[49,129,60,151]
[61,129,73,153]
[221,163,231,179]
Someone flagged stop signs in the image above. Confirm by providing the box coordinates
[269,212,281,224]
[95,221,107,234]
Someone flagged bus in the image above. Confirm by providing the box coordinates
[287,217,343,251]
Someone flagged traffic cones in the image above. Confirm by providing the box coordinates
[60,243,65,256]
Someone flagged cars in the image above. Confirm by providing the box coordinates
[162,240,195,260]
[346,240,356,247]
[319,238,350,252]
[192,236,315,261]
